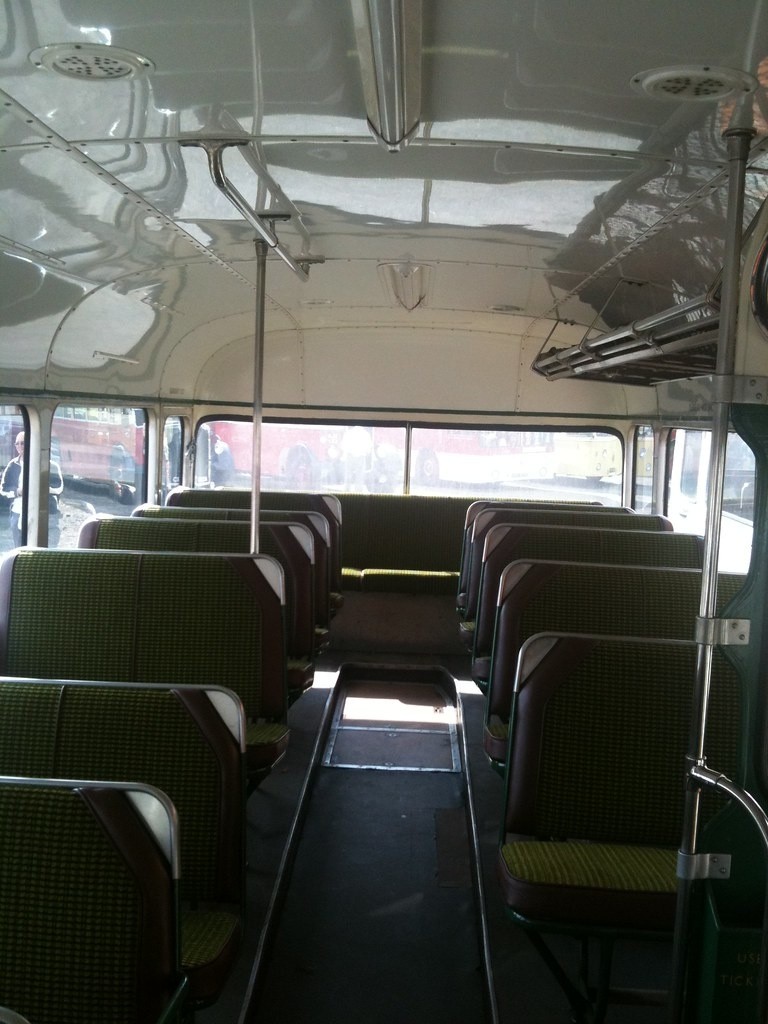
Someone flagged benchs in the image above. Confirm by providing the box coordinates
[0,489,768,1023]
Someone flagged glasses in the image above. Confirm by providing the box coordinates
[14,442,25,446]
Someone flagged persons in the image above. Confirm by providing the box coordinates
[0,431,63,548]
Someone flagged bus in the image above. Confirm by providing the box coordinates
[669,430,756,574]
[0,405,212,505]
[209,420,654,491]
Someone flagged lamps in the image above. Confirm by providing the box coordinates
[354,0,422,153]
